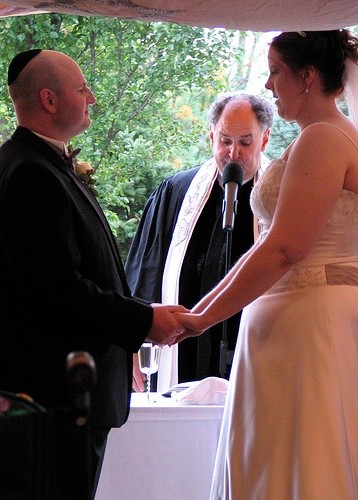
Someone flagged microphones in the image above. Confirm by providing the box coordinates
[222,162,243,232]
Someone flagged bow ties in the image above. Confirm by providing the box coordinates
[65,146,82,169]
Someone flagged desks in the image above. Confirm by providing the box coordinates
[95,392,224,500]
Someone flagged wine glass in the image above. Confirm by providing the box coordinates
[137,342,161,404]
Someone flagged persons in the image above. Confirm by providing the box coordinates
[0,49,192,500]
[174,29,358,500]
[125,92,275,393]
